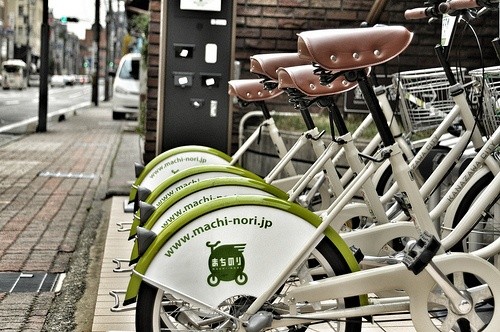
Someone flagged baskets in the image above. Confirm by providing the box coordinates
[470,65,500,145]
[391,67,466,133]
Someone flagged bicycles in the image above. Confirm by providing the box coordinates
[123,0,500,332]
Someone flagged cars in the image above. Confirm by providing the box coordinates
[107,53,141,120]
[28,75,40,87]
[50,73,92,88]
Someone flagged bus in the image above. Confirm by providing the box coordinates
[1,58,29,90]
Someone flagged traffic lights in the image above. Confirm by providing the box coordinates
[60,16,78,23]
